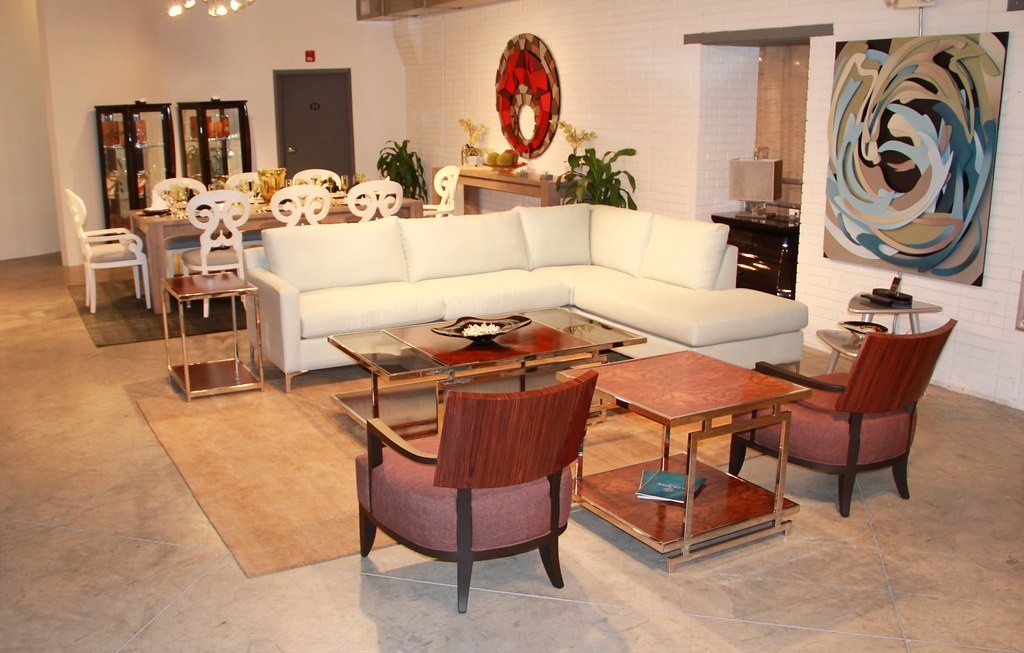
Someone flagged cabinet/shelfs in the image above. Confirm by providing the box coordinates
[178,99,252,195]
[712,210,801,301]
[94,100,176,244]
[556,351,812,573]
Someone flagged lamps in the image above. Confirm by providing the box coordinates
[728,146,783,220]
[167,0,255,18]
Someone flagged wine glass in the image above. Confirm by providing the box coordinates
[158,173,364,219]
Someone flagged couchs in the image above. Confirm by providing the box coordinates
[240,203,808,396]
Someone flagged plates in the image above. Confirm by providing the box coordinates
[249,199,265,204]
[144,206,171,215]
[431,314,532,342]
[341,200,362,205]
[262,205,295,211]
[329,193,345,198]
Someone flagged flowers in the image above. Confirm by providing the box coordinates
[549,118,598,157]
[456,118,487,166]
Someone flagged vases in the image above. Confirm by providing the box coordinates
[467,156,481,165]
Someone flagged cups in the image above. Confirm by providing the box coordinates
[257,168,286,205]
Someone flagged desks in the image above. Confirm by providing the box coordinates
[128,195,423,315]
[458,168,580,216]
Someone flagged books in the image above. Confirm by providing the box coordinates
[635,470,707,503]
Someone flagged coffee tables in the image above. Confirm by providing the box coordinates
[159,270,265,403]
[816,290,942,397]
[326,305,647,431]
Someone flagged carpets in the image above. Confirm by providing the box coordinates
[135,351,764,579]
[66,279,247,347]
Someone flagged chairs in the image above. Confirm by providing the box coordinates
[727,317,958,518]
[354,369,598,614]
[65,166,463,318]
